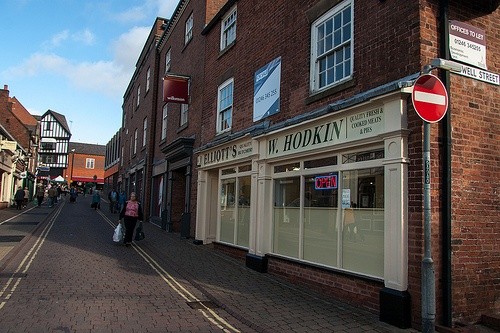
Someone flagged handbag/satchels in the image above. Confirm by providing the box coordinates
[135,223,144,240]
[112,223,123,242]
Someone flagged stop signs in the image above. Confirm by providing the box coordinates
[412,74,449,124]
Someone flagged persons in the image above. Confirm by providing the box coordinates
[15,186,25,211]
[56,185,102,211]
[108,186,118,215]
[118,190,127,213]
[24,187,29,208]
[119,192,142,248]
[36,183,45,208]
[48,184,58,208]
